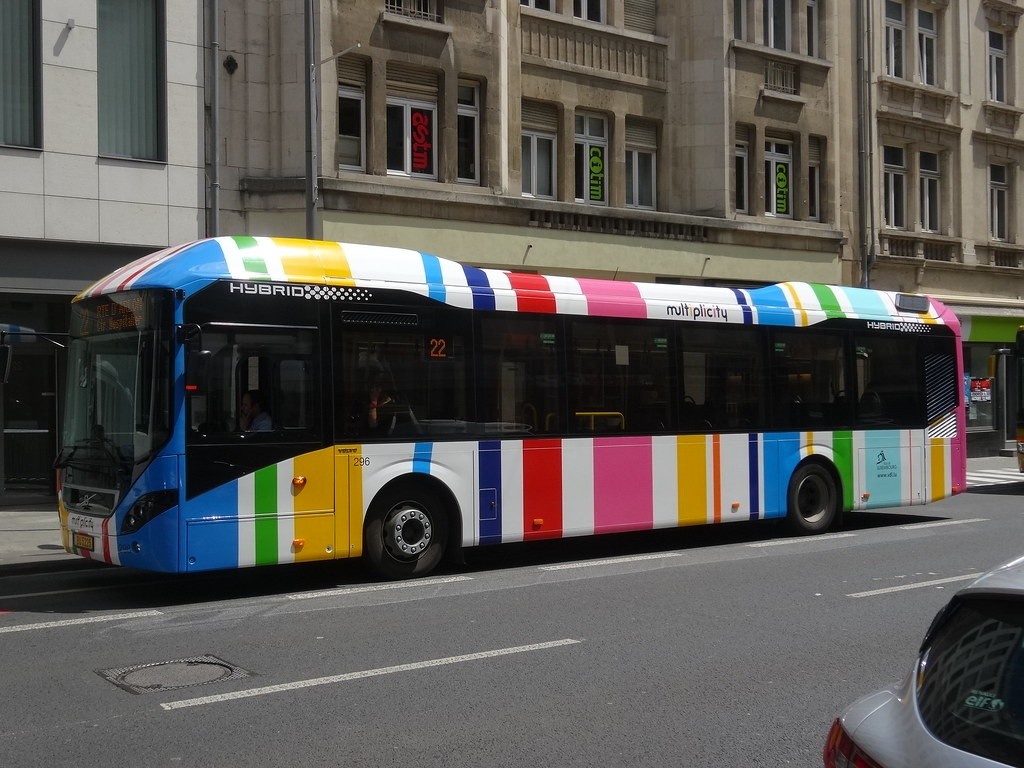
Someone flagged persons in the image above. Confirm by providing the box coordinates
[345,373,394,434]
[239,389,272,431]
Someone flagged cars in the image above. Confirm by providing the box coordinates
[823,552,1024,768]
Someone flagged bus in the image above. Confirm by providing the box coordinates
[53,236,969,584]
[1012,324,1024,472]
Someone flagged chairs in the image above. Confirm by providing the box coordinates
[859,390,883,417]
[636,417,755,431]
